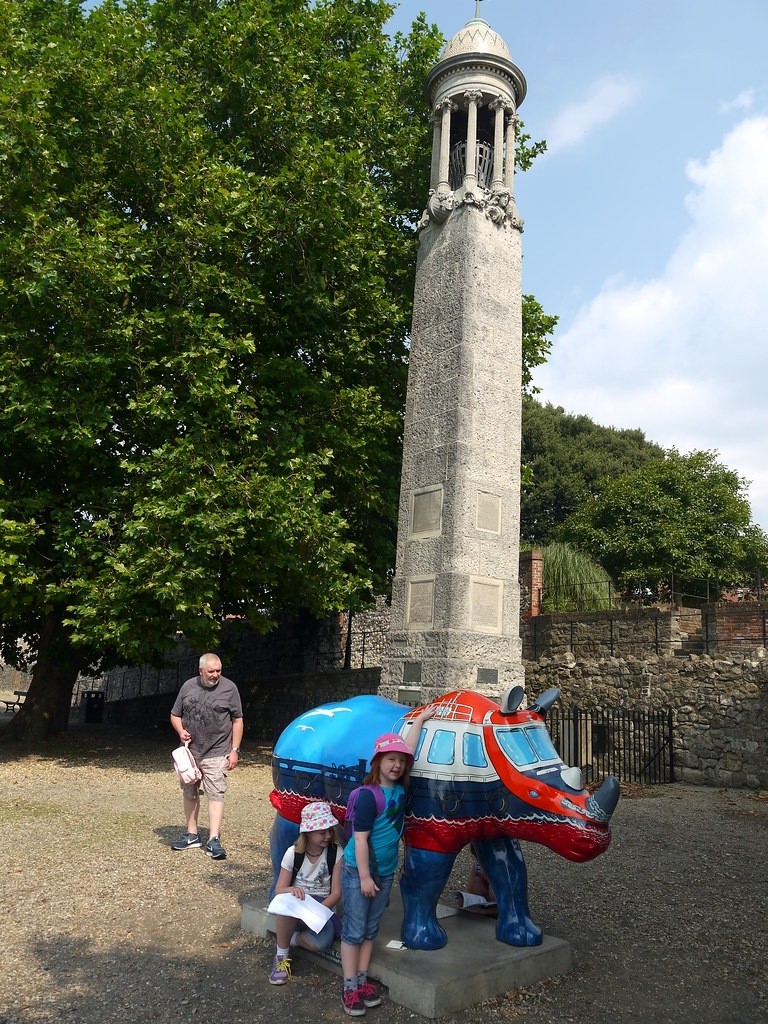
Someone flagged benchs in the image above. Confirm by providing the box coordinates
[1,691,28,714]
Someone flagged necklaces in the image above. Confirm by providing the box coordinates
[305,845,324,857]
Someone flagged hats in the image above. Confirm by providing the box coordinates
[299,802,339,833]
[370,733,414,770]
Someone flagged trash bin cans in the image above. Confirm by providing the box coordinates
[77,690,105,725]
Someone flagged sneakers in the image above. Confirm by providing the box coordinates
[205,836,227,859]
[171,830,203,850]
[358,981,383,1007]
[269,955,292,985]
[341,987,366,1016]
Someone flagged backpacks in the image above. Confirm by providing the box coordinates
[172,739,202,785]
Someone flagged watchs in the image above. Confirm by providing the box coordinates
[231,747,242,756]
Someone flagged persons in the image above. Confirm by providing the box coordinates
[268,801,346,985]
[339,704,437,1017]
[170,652,244,860]
